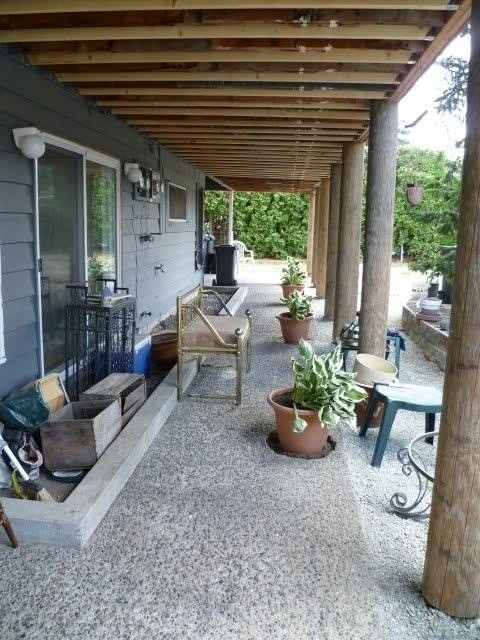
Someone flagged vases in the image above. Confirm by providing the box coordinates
[151,333,178,363]
[354,354,399,428]
[420,297,442,316]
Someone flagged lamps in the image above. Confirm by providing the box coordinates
[11,127,46,159]
[124,162,143,183]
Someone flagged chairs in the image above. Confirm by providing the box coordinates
[229,240,255,274]
[175,283,252,405]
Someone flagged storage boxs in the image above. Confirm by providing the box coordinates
[77,372,148,421]
[93,335,152,383]
[39,399,124,471]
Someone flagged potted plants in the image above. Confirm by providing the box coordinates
[280,256,307,298]
[277,289,314,343]
[267,339,368,453]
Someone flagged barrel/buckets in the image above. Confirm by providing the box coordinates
[353,353,399,429]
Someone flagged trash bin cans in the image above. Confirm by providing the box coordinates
[212,244,236,286]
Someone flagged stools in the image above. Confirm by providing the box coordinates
[339,323,402,377]
[359,386,444,468]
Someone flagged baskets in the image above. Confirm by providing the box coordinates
[94,342,152,382]
